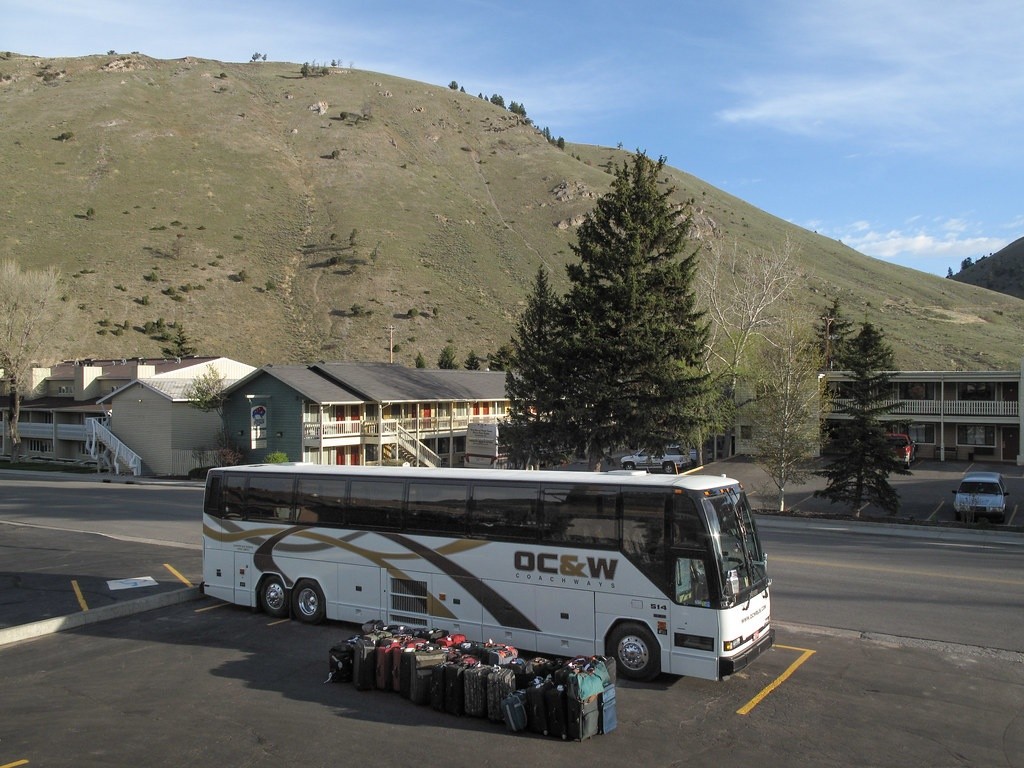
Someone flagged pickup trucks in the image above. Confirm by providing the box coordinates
[883,433,916,469]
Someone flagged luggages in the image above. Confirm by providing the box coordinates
[330,620,617,742]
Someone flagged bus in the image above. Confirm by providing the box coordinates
[464,422,567,471]
[200,462,776,683]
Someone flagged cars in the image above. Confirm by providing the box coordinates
[952,472,1010,524]
[620,445,692,474]
